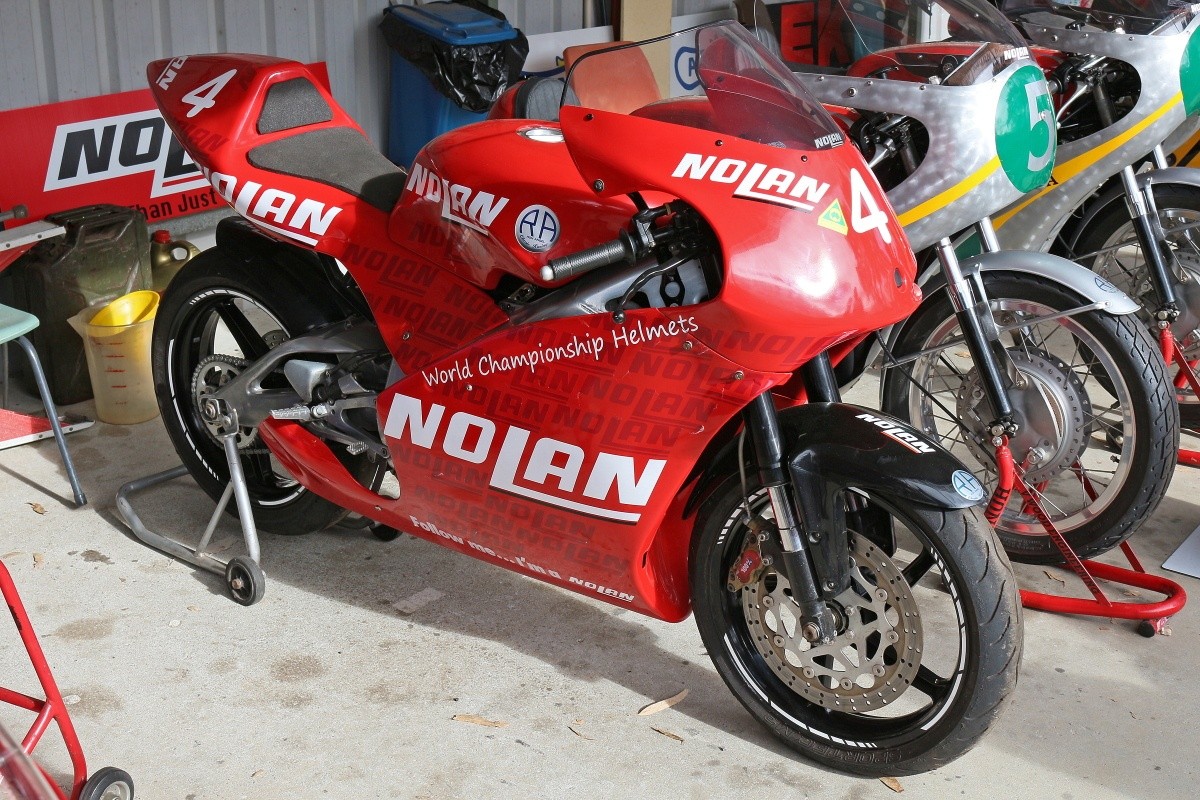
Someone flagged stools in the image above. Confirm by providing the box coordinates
[0,304,88,506]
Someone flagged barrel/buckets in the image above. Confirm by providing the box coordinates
[13,203,152,407]
[151,230,202,296]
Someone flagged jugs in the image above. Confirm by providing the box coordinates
[68,298,161,425]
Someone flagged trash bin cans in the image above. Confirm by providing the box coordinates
[382,1,518,174]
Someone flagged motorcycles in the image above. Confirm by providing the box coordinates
[379,2,1181,572]
[146,16,1028,779]
[628,0,1200,432]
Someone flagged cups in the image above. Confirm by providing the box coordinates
[84,289,161,361]
